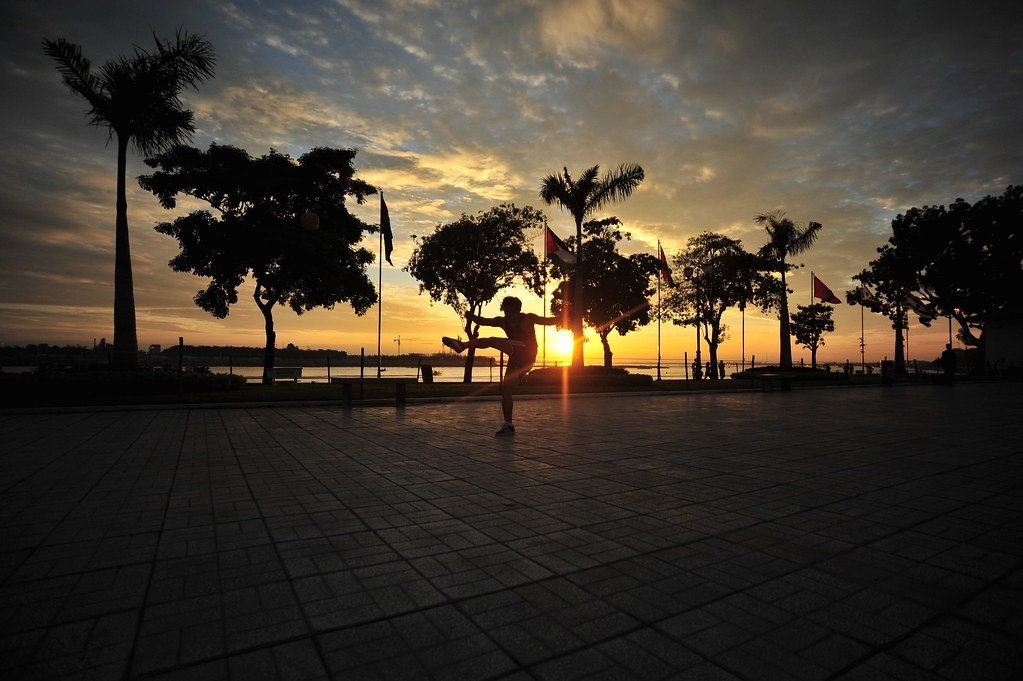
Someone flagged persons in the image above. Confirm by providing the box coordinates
[843,360,854,375]
[987,360,1000,376]
[826,364,831,371]
[719,360,725,380]
[442,296,562,435]
[704,362,711,379]
[866,365,874,374]
[938,344,958,387]
[692,358,703,379]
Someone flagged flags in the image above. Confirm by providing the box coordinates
[863,284,876,302]
[381,192,393,266]
[903,293,932,317]
[661,247,675,288]
[814,275,842,304]
[546,226,586,269]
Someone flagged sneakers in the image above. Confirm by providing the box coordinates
[442,336,466,353]
[496,424,515,436]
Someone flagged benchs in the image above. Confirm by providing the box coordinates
[330,377,419,410]
[273,366,303,382]
